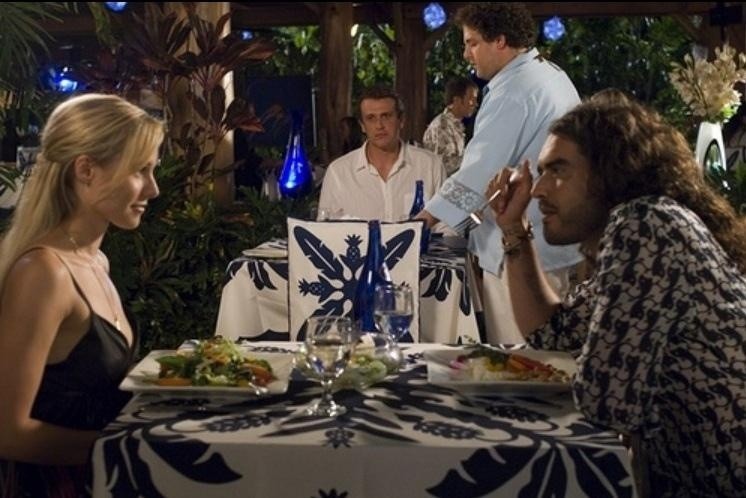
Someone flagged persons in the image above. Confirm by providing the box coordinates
[2,92,166,498]
[484,90,746,498]
[233,97,280,202]
[315,88,466,240]
[315,115,364,169]
[412,1,596,348]
[422,80,482,174]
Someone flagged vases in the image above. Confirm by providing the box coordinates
[694,121,727,189]
[276,106,315,196]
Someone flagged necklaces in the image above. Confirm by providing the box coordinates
[59,221,121,328]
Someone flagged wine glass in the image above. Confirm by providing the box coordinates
[372,283,417,347]
[301,317,358,419]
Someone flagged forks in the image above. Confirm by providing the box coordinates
[451,174,516,236]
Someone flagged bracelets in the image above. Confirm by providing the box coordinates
[320,144,329,152]
[501,220,537,256]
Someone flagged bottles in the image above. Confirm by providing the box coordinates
[410,180,432,253]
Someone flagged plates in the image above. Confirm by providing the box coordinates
[423,348,577,397]
[118,349,296,401]
[242,247,288,259]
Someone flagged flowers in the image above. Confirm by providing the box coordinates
[664,39,745,122]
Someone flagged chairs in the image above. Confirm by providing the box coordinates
[283,216,427,344]
